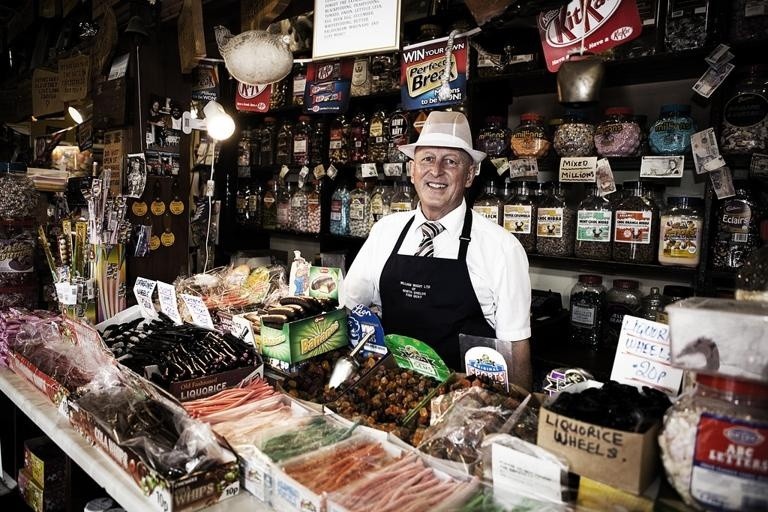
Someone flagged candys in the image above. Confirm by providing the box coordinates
[290,188,389,237]
[478,118,697,160]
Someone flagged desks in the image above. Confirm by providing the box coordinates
[0,321,280,512]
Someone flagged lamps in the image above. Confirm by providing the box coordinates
[66,98,95,122]
[180,100,237,143]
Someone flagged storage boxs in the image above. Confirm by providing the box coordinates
[534,393,666,500]
[14,468,100,512]
[21,435,78,489]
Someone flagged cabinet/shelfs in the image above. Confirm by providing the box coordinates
[225,148,768,284]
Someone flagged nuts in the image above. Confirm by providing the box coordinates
[283,348,524,464]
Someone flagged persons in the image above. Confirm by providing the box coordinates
[339,113,532,393]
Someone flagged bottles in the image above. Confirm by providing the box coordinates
[349,55,400,95]
[228,105,416,164]
[474,103,699,157]
[567,276,695,345]
[271,73,306,108]
[406,23,469,44]
[709,1,767,272]
[656,367,766,509]
[472,177,704,267]
[233,178,422,239]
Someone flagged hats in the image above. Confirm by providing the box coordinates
[397,109,489,168]
